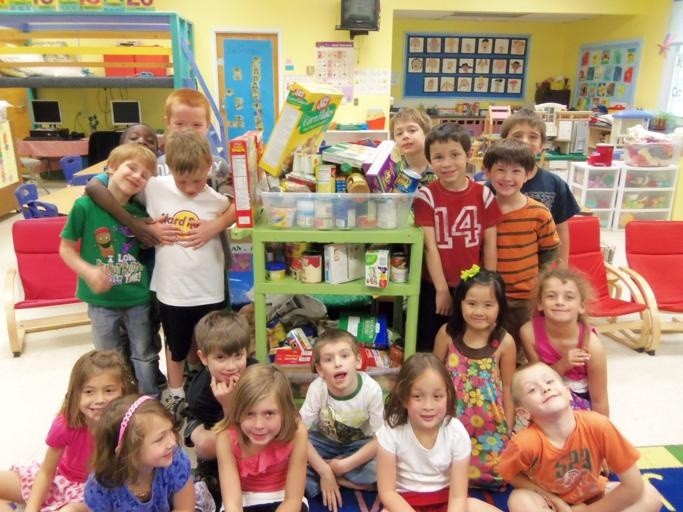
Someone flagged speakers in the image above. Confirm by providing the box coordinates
[340,0,381,30]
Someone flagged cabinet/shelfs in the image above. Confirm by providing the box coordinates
[248,225,424,408]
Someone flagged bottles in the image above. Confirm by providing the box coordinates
[596,144,614,166]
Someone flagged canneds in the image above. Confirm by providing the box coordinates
[266,318,287,346]
[336,201,355,231]
[301,251,323,284]
[346,173,371,204]
[297,200,315,229]
[391,252,408,283]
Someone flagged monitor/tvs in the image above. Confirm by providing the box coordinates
[110,99,143,133]
[31,99,63,131]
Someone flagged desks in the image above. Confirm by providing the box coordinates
[18,137,89,176]
[73,159,108,176]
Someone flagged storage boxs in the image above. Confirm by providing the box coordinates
[256,179,419,230]
[568,140,681,231]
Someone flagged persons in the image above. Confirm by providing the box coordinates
[299,330,383,512]
[433,265,518,491]
[84,395,216,511]
[185,311,259,464]
[520,265,608,419]
[392,107,579,368]
[211,362,311,512]
[376,353,505,512]
[0,349,137,511]
[59,88,235,430]
[498,360,663,511]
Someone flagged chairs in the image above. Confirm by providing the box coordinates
[59,155,83,188]
[4,184,93,357]
[550,217,683,355]
[20,158,51,195]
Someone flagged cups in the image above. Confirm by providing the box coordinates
[586,155,605,166]
